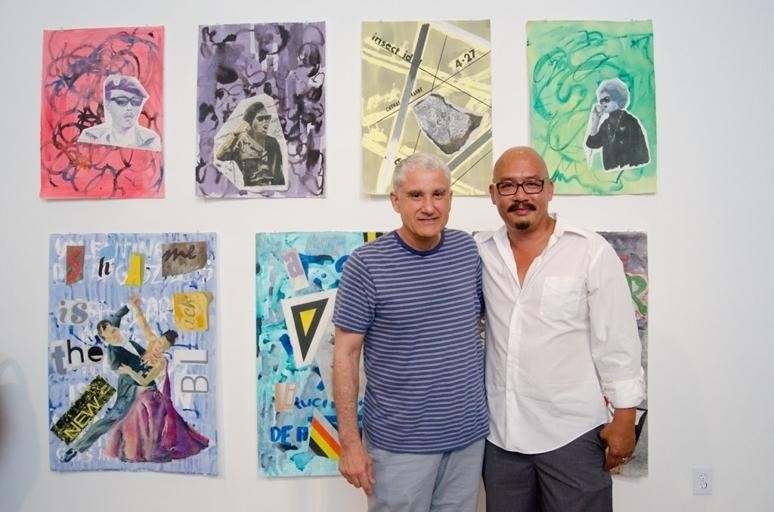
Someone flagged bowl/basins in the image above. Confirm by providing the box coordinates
[607,112,624,144]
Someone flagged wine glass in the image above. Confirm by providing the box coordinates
[622,457,628,462]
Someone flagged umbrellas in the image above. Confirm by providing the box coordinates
[64,449,76,462]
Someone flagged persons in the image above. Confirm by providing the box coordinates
[466,146,646,512]
[58,292,210,464]
[217,101,285,187]
[74,72,160,151]
[330,153,481,512]
[583,80,649,169]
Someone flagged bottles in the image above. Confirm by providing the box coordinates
[106,76,147,97]
[243,102,264,124]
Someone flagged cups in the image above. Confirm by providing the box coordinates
[600,96,609,103]
[256,115,271,121]
[111,95,142,106]
[496,179,545,195]
